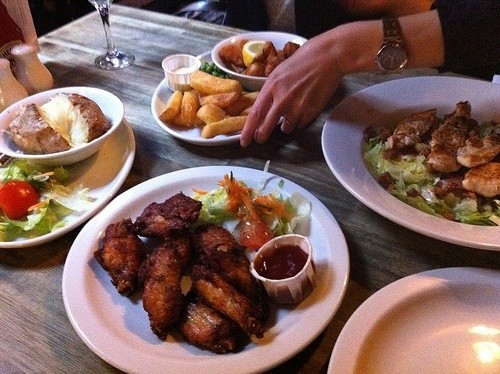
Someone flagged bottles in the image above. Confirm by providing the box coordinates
[0,59,29,113]
[11,44,53,96]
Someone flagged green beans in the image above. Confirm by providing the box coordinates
[199,62,228,80]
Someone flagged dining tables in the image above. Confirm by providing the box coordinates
[1,4,500,374]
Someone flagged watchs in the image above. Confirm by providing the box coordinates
[375,15,408,74]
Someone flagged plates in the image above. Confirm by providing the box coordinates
[321,76,500,251]
[150,50,284,146]
[0,117,136,248]
[327,267,500,374]
[62,165,349,374]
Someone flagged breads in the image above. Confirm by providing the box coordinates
[7,92,109,155]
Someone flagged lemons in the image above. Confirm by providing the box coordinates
[243,39,266,67]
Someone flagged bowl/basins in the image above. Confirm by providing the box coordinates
[211,32,308,91]
[0,86,124,166]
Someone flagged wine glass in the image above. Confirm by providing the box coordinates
[88,0,135,70]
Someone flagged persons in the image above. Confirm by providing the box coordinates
[240,0,500,147]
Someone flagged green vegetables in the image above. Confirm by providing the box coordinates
[365,119,500,226]
[188,170,310,250]
[0,157,93,242]
